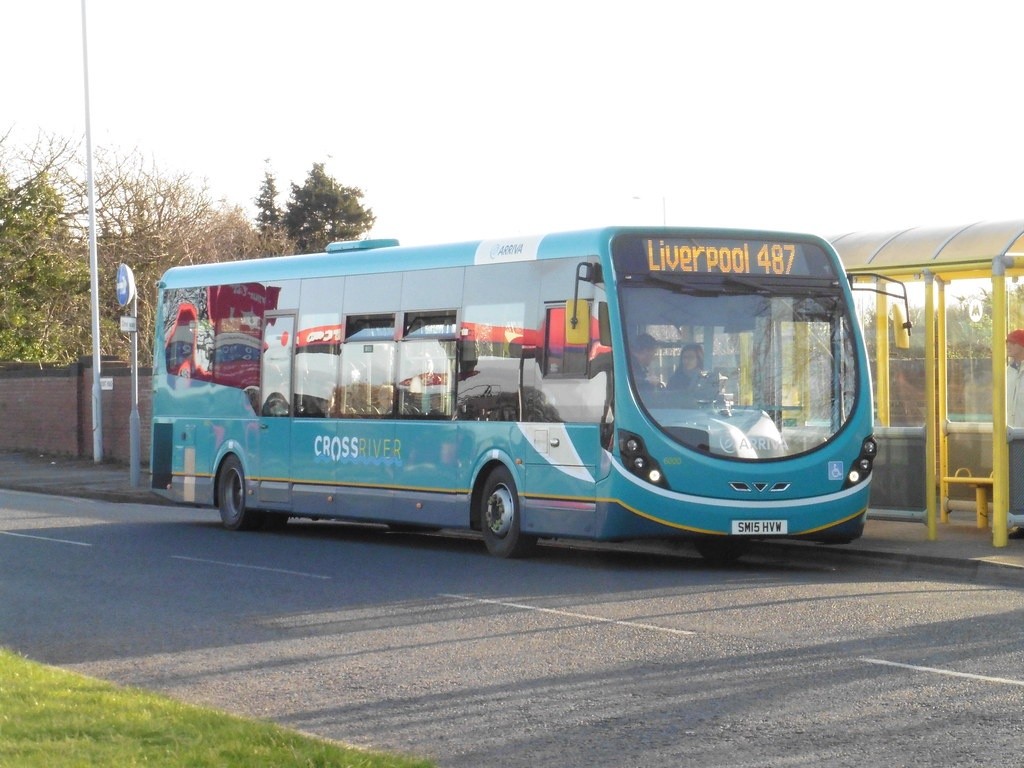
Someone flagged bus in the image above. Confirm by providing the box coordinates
[149,223,913,559]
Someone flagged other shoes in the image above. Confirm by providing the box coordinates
[1009,527,1024,539]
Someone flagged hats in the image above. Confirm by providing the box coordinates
[1006,329,1024,347]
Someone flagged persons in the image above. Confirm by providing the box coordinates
[1005,329,1024,539]
[376,385,393,415]
[668,343,709,410]
[628,334,661,410]
